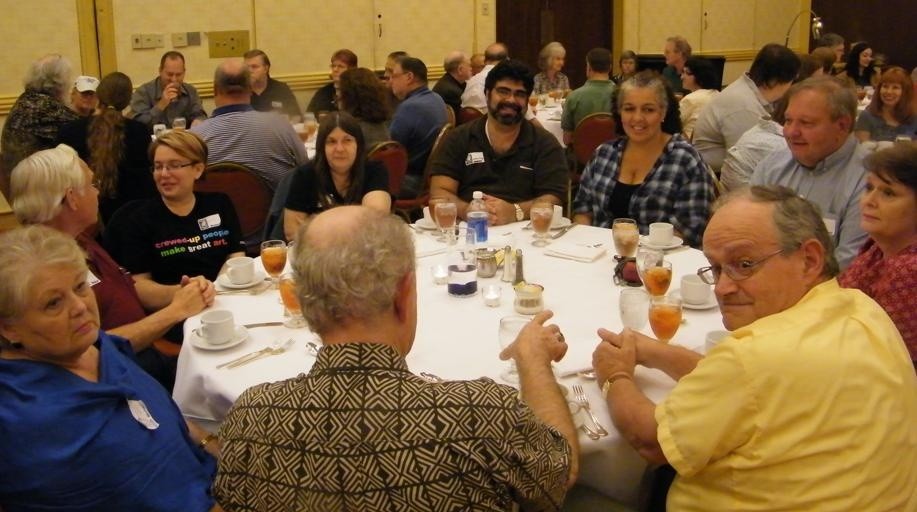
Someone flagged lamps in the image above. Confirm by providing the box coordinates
[784,8,825,49]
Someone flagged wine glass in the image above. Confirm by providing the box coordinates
[856,84,874,107]
[497,316,533,384]
[281,112,327,160]
[261,239,309,328]
[414,190,576,314]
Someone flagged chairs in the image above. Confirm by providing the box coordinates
[392,123,453,224]
[368,140,408,213]
[193,163,273,256]
[458,107,483,121]
[708,165,721,197]
[447,104,456,128]
[566,112,617,218]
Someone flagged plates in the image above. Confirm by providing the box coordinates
[189,325,250,351]
[219,272,265,289]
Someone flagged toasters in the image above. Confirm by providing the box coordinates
[526,89,573,121]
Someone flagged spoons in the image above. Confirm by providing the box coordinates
[577,371,596,380]
[304,341,319,356]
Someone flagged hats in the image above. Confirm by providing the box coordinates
[71,75,101,93]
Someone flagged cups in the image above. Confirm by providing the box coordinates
[196,310,235,343]
[609,213,718,344]
[225,256,255,284]
[861,135,912,151]
[675,93,683,100]
[172,118,185,132]
[152,125,165,138]
[170,83,180,103]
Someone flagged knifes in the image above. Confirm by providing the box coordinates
[244,322,282,328]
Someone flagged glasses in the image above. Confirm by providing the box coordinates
[491,83,528,101]
[61,177,102,204]
[697,243,803,287]
[149,161,199,174]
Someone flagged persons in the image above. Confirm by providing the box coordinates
[1,33,916,512]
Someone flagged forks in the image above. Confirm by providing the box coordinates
[572,385,609,438]
[216,333,296,369]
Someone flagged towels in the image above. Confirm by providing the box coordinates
[553,346,593,379]
[411,232,446,257]
[545,225,613,264]
